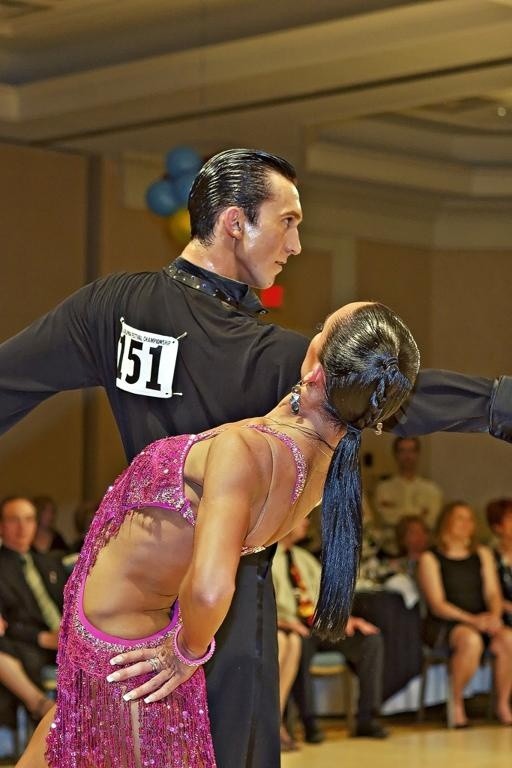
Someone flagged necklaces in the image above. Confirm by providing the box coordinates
[263,413,339,462]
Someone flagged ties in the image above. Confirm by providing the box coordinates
[21,552,62,629]
[286,549,314,628]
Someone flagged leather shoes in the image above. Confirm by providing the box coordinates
[356,721,390,739]
[305,730,324,742]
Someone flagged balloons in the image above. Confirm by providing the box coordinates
[144,144,202,249]
[260,285,285,309]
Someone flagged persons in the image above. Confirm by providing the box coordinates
[0,490,106,725]
[267,434,512,754]
[0,143,512,768]
[12,300,422,768]
